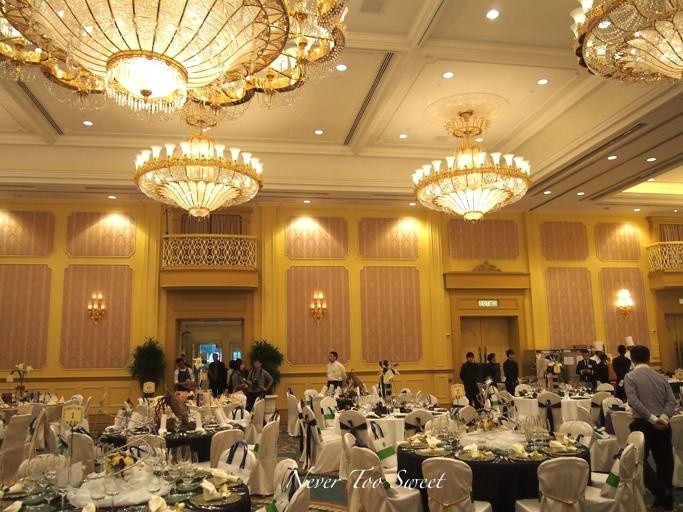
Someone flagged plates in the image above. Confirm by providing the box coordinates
[413,441,579,462]
[192,492,241,509]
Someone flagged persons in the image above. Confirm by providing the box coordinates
[193,352,274,411]
[502,349,520,396]
[460,347,481,412]
[482,352,502,389]
[173,356,195,391]
[574,349,610,389]
[323,350,348,391]
[610,344,631,403]
[623,344,677,512]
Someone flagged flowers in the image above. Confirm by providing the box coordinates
[376,360,401,384]
[9,364,34,398]
[193,357,208,392]
[544,356,564,374]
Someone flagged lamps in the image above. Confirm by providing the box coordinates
[615,289,633,320]
[410,109,535,224]
[134,114,265,223]
[87,290,105,326]
[0,0,347,123]
[569,0,683,83]
[310,290,329,327]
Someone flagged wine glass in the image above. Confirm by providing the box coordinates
[427,414,551,459]
[21,445,199,512]
